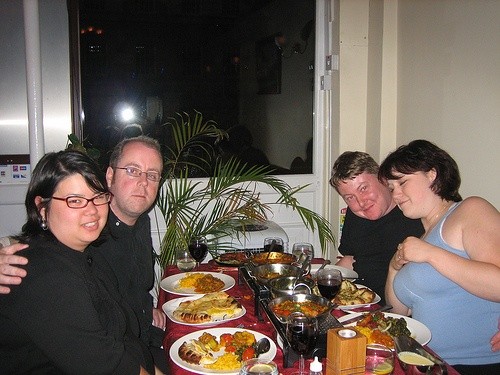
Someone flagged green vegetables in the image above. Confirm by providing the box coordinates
[231,342,260,363]
[290,302,320,317]
[373,315,412,339]
[272,279,313,290]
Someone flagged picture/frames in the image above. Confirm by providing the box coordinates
[254,33,283,95]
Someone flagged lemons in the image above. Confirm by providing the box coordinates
[373,362,393,375]
[250,363,275,373]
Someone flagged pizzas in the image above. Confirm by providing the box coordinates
[313,280,376,307]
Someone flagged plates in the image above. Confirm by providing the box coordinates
[337,312,432,351]
[162,296,247,327]
[213,256,244,267]
[331,284,381,309]
[297,264,358,285]
[159,271,236,296]
[169,328,277,375]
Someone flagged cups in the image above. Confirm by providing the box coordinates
[264,237,283,253]
[362,345,394,375]
[175,249,196,272]
[292,243,314,264]
[240,358,279,375]
[317,269,342,301]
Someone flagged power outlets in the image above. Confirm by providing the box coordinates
[318,72,334,93]
[323,54,342,71]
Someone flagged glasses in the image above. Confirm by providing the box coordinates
[116,165,161,181]
[41,191,112,209]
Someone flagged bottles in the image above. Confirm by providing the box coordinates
[309,357,323,375]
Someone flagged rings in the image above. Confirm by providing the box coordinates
[399,256,400,259]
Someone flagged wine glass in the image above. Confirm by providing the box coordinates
[285,314,318,375]
[188,236,208,271]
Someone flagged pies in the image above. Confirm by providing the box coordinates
[178,331,220,364]
[358,311,386,328]
[194,274,225,293]
[173,291,243,324]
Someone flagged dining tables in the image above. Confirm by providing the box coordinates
[159,250,462,375]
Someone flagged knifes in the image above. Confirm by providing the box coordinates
[341,305,393,325]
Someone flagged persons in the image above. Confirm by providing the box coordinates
[0,133,172,375]
[329,151,500,352]
[376,139,500,375]
[0,149,152,375]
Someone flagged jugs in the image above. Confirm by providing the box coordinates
[394,336,448,375]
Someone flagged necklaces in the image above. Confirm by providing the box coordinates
[434,200,451,219]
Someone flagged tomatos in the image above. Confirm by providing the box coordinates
[220,333,255,360]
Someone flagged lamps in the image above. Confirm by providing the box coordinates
[268,21,313,60]
[235,56,249,71]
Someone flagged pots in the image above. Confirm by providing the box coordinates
[263,276,317,297]
[268,283,333,324]
[247,264,309,285]
[251,252,296,266]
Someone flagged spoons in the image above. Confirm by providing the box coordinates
[254,338,270,358]
